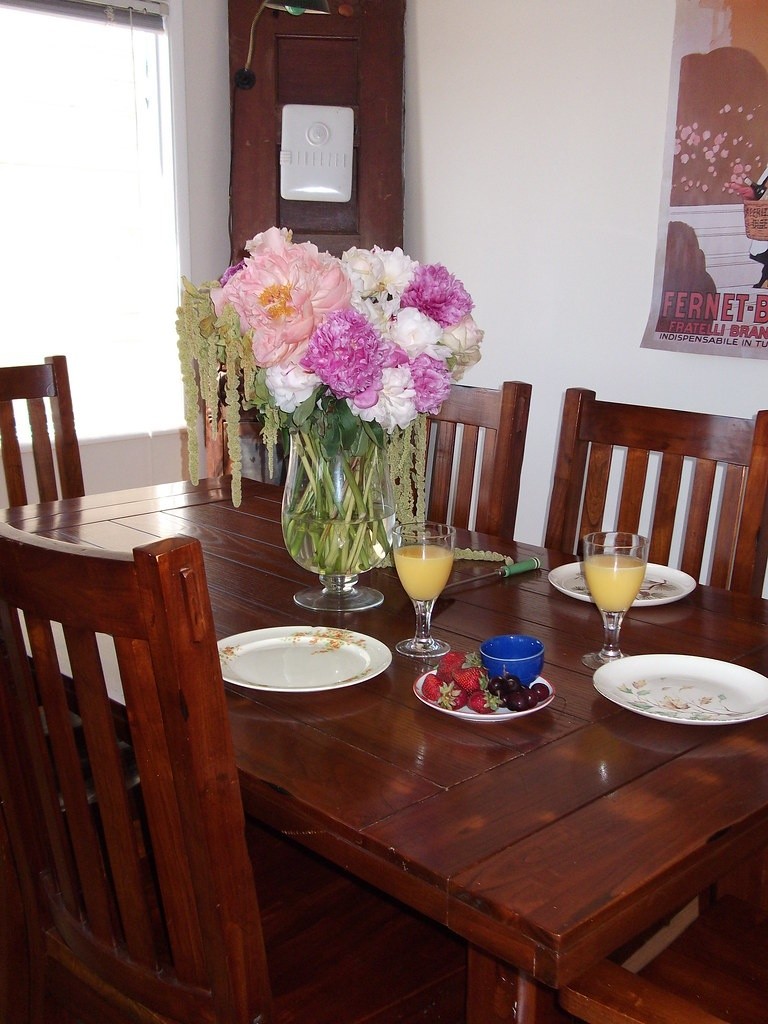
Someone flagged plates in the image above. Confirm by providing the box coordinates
[217,625,392,692]
[414,666,556,722]
[548,561,696,606]
[593,653,768,725]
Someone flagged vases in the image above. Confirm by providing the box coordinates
[279,423,397,613]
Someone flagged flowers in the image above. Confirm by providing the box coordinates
[175,227,485,575]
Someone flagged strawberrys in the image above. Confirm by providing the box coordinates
[422,650,503,714]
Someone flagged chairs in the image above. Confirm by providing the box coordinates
[544,387,768,600]
[0,518,467,1024]
[388,381,534,539]
[0,355,85,707]
[205,372,291,488]
[557,839,768,1024]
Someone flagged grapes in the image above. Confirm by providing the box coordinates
[489,675,548,710]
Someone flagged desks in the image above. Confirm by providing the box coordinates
[0,475,768,1024]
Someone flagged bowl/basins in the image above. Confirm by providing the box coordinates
[480,634,544,687]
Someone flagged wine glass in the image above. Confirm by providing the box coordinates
[584,532,649,670]
[391,522,457,658]
